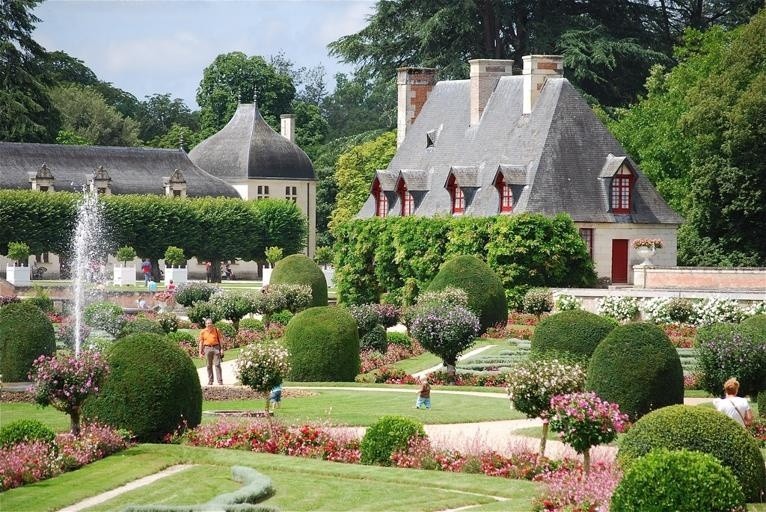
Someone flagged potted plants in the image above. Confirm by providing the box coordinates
[113,244,138,289]
[163,246,189,290]
[4,240,31,284]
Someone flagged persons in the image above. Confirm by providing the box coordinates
[270,385,281,409]
[717,378,752,429]
[199,317,224,385]
[224,264,233,280]
[415,374,432,409]
[142,258,152,287]
[148,278,157,291]
[205,261,211,283]
[167,280,176,295]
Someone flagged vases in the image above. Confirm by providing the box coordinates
[636,246,658,266]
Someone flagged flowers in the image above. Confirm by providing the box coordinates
[630,236,663,251]
